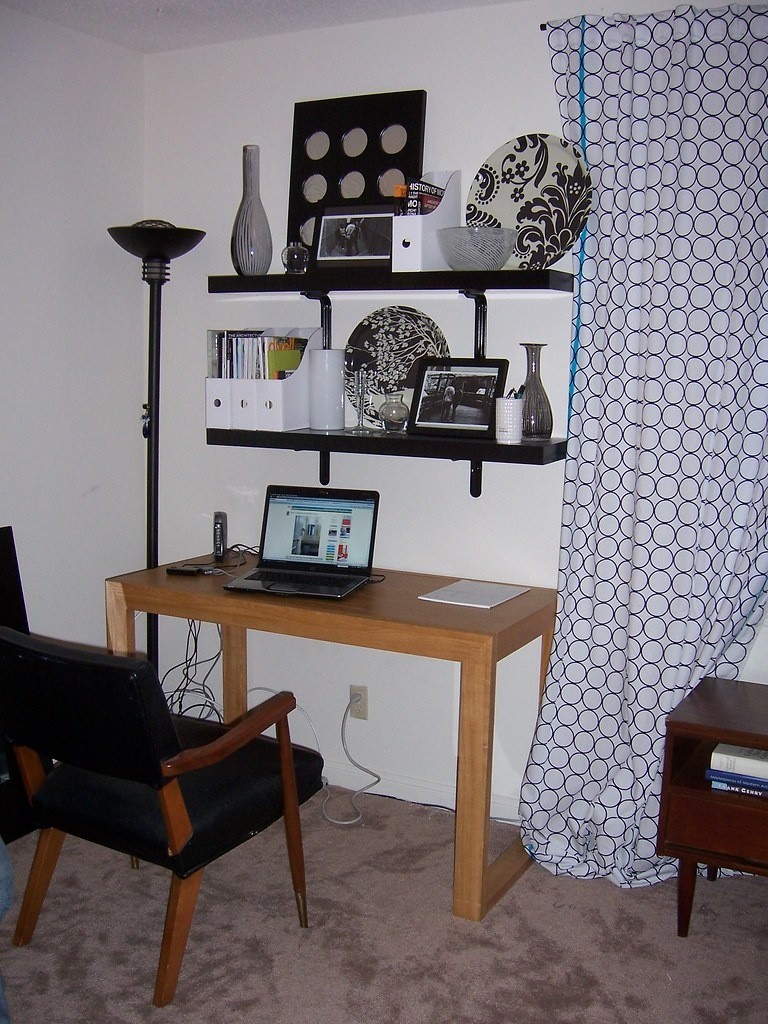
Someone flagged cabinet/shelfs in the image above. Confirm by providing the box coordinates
[653,677,768,939]
[205,267,575,499]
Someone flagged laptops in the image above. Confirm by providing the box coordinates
[223,485,381,601]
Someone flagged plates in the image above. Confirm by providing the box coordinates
[465,134,593,270]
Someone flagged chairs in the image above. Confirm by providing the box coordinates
[0,626,324,1008]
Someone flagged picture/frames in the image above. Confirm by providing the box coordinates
[308,204,403,269]
[405,357,508,440]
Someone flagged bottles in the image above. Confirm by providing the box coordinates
[379,393,409,431]
[231,145,272,275]
[308,349,346,430]
[281,242,310,274]
[519,343,553,442]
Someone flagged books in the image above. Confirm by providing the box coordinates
[705,743,768,798]
[393,180,445,216]
[207,329,308,380]
[418,580,530,609]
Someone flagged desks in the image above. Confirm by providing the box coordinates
[102,547,557,922]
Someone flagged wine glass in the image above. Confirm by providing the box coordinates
[351,371,370,434]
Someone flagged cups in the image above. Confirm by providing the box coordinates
[496,398,523,444]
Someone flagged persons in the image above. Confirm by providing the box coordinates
[339,220,359,255]
[441,381,462,422]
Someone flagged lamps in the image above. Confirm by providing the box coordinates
[106,219,207,675]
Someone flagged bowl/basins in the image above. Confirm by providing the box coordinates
[437,227,518,271]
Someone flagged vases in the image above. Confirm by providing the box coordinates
[518,343,553,442]
[230,145,274,275]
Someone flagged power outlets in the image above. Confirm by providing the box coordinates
[350,685,369,721]
